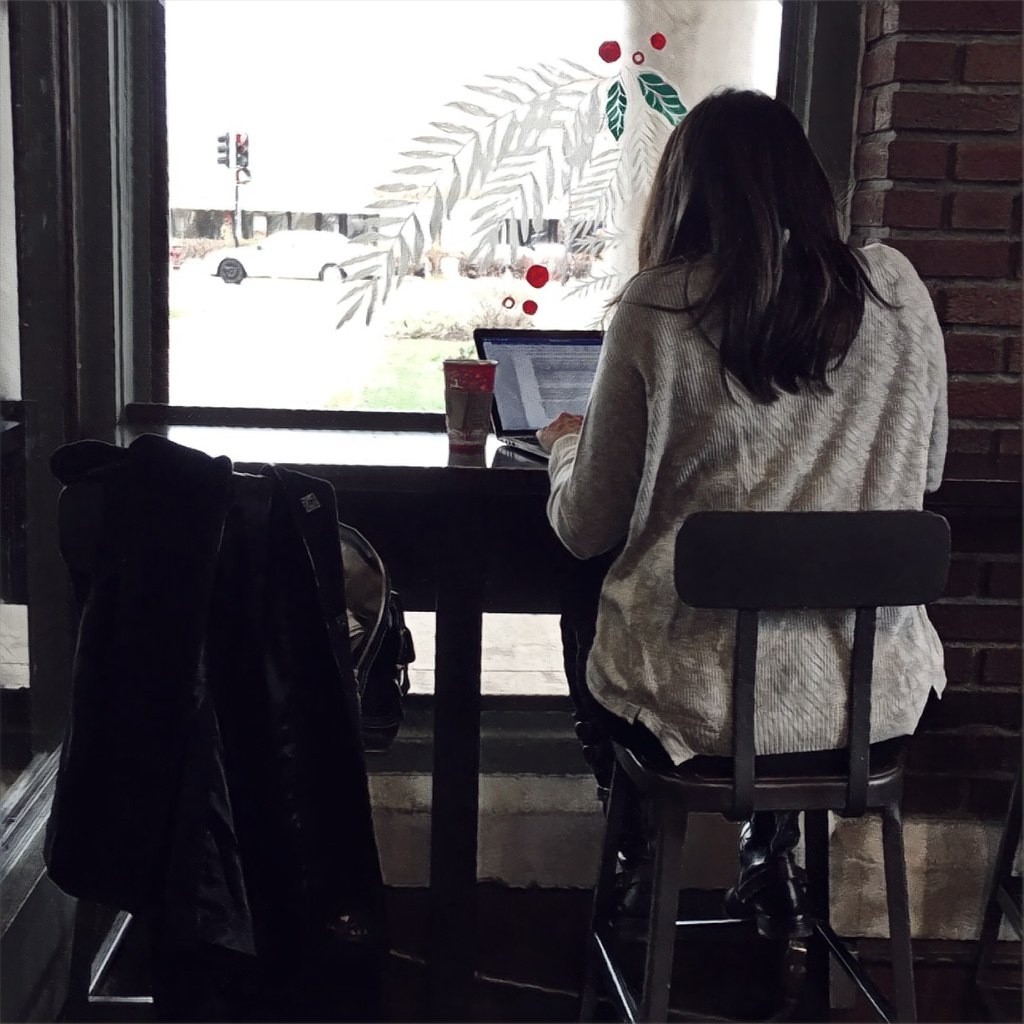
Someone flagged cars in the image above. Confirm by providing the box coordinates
[202,231,385,285]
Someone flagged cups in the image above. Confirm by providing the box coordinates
[442,357,499,454]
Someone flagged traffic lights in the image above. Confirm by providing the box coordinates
[236,133,248,167]
[218,133,229,167]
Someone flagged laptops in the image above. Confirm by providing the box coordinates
[473,329,606,461]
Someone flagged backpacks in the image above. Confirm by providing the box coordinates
[338,519,414,752]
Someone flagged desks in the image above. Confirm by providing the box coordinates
[93,416,568,1022]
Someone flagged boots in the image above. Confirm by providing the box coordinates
[721,808,814,940]
[586,785,664,961]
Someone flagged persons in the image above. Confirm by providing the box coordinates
[536,89,949,941]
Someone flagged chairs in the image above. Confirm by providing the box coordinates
[582,510,953,1024]
[75,470,266,1013]
